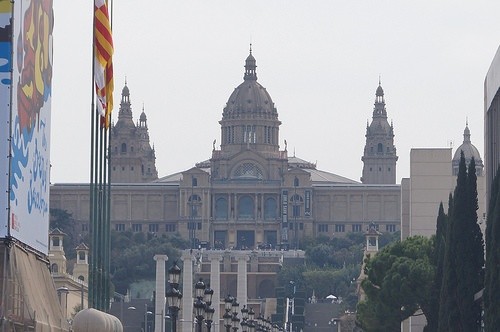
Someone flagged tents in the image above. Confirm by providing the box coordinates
[326,295,337,299]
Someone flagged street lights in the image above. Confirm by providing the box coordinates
[193,277,206,332]
[223,295,233,332]
[57,283,84,310]
[165,261,183,332]
[205,287,215,332]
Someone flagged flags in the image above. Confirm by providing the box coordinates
[91,0,115,130]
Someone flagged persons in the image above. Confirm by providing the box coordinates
[305,321,318,328]
[332,300,336,304]
[329,320,336,327]
